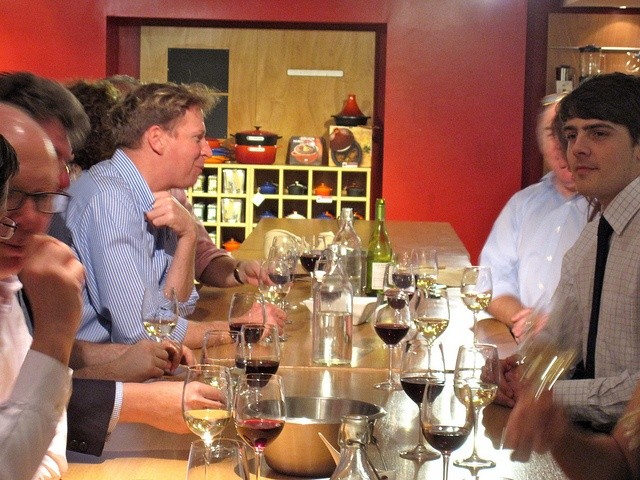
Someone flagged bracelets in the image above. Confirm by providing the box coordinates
[233,261,244,285]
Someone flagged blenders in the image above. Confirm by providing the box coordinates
[579,44,606,84]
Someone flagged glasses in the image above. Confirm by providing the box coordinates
[0,214,18,240]
[7,190,72,214]
[539,92,569,106]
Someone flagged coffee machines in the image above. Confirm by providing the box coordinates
[555,63,575,95]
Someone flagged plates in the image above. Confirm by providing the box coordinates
[300,296,387,325]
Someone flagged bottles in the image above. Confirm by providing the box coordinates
[366,199,392,296]
[331,416,379,480]
[312,256,353,366]
[329,208,363,296]
[315,244,345,287]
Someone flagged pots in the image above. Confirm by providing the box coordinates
[285,181,307,195]
[345,181,364,196]
[331,114,371,124]
[228,143,284,164]
[313,182,333,196]
[204,154,230,163]
[206,138,224,148]
[291,144,319,164]
[329,128,362,167]
[316,210,335,219]
[230,126,283,146]
[286,210,306,219]
[259,210,276,220]
[353,211,364,220]
[222,238,242,252]
[259,180,277,194]
[211,148,230,156]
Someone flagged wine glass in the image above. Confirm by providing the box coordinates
[229,291,266,360]
[267,246,295,312]
[182,365,234,480]
[233,373,288,480]
[420,382,475,480]
[390,250,413,304]
[460,266,493,352]
[624,51,640,74]
[187,439,248,480]
[372,292,411,391]
[412,248,439,292]
[313,230,336,251]
[258,260,291,342]
[400,343,446,459]
[272,234,299,269]
[236,323,280,402]
[300,236,326,301]
[141,286,178,343]
[453,344,499,469]
[201,330,243,389]
[409,283,450,380]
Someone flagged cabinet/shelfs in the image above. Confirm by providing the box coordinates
[184,163,371,251]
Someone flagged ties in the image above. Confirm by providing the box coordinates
[585,212,614,378]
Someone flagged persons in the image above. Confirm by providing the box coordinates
[36,81,289,366]
[502,384,639,479]
[72,337,183,387]
[1,132,20,240]
[478,93,600,343]
[0,100,88,480]
[60,78,126,182]
[99,74,285,287]
[481,72,640,425]
[1,70,231,458]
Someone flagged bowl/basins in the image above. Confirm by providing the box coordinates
[256,396,388,476]
